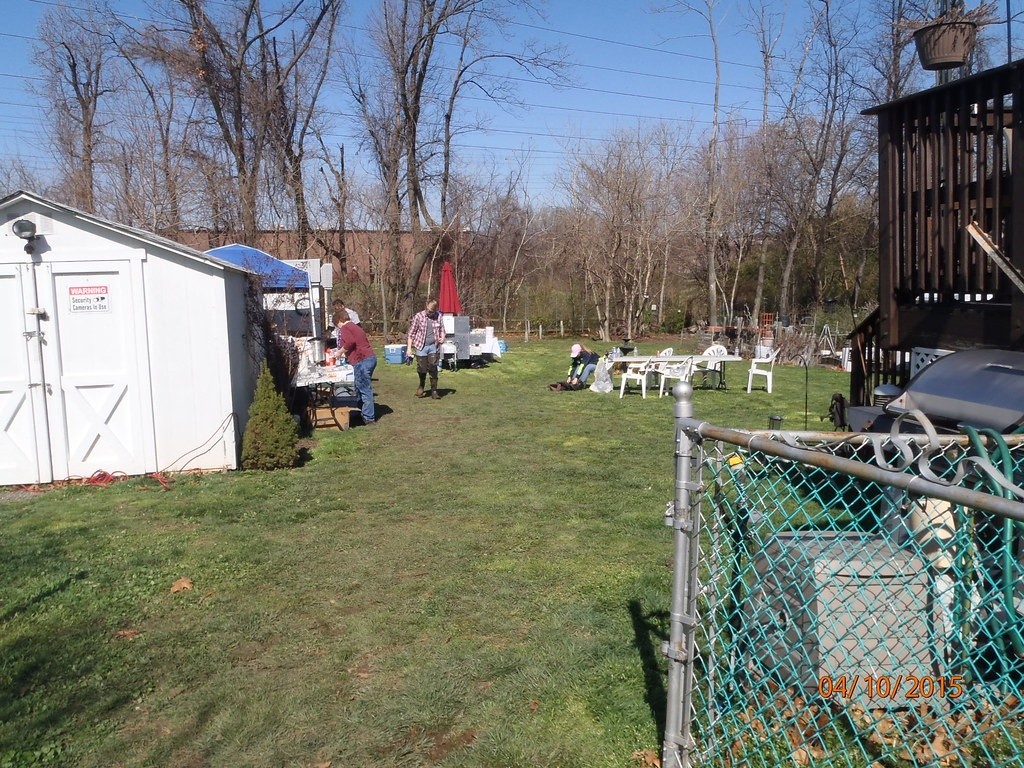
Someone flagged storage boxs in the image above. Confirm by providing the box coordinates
[740,529,945,709]
[383,343,407,364]
[332,384,358,407]
[303,406,362,431]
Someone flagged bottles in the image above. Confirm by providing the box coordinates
[469,324,471,332]
[735,347,739,357]
[325,347,346,366]
[616,347,620,357]
[612,347,617,358]
[656,349,660,357]
[633,346,638,358]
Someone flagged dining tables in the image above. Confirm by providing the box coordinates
[296,363,354,432]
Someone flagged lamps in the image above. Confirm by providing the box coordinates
[768,415,784,430]
[11,218,43,255]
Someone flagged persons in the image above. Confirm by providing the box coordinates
[324,298,362,347]
[567,343,600,389]
[405,297,447,400]
[332,307,378,425]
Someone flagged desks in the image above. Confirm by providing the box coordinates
[612,355,743,394]
[846,405,1024,550]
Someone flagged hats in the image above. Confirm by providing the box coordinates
[570,343,582,358]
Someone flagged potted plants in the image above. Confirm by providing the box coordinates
[879,0,1000,71]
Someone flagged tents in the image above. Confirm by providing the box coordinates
[202,242,318,373]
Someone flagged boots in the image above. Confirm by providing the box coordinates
[429,353,441,400]
[416,356,428,398]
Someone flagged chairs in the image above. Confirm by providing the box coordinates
[747,348,781,393]
[619,344,727,399]
[600,351,615,382]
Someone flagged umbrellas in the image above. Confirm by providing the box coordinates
[438,261,465,316]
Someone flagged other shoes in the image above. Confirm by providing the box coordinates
[353,418,375,426]
